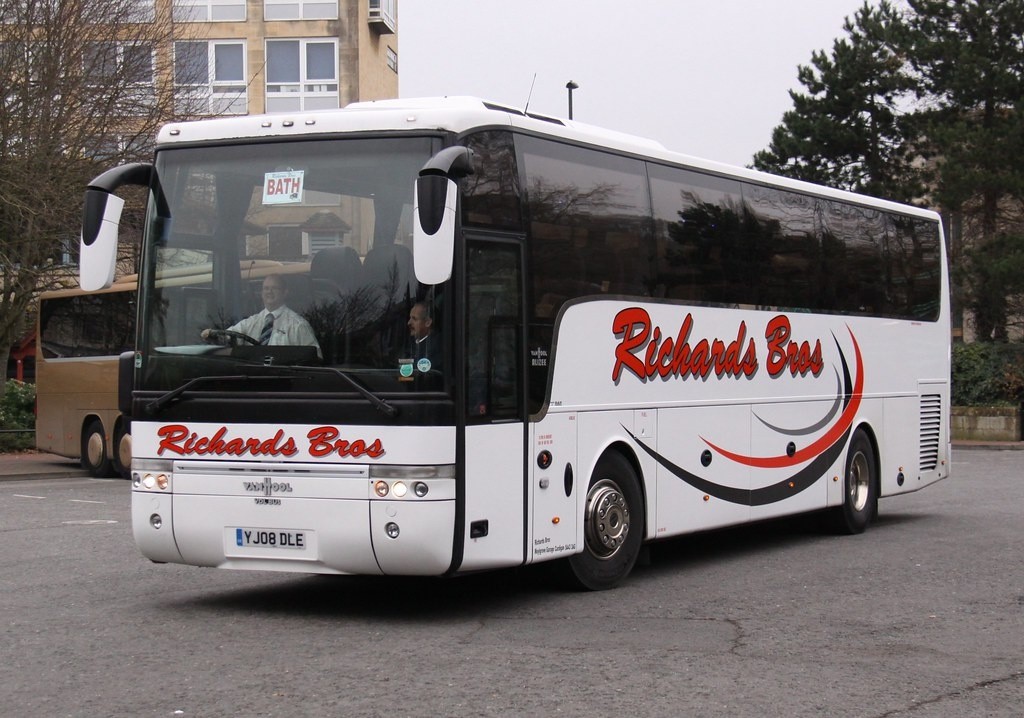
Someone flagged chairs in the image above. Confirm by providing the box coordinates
[290,279,350,369]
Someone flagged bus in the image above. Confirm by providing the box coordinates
[76,93,955,595]
[34,257,371,483]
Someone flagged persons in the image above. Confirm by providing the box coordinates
[201,272,324,359]
[398,300,444,369]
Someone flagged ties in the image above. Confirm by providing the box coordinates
[259,313,274,345]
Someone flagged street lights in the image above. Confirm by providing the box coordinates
[566,81,579,120]
